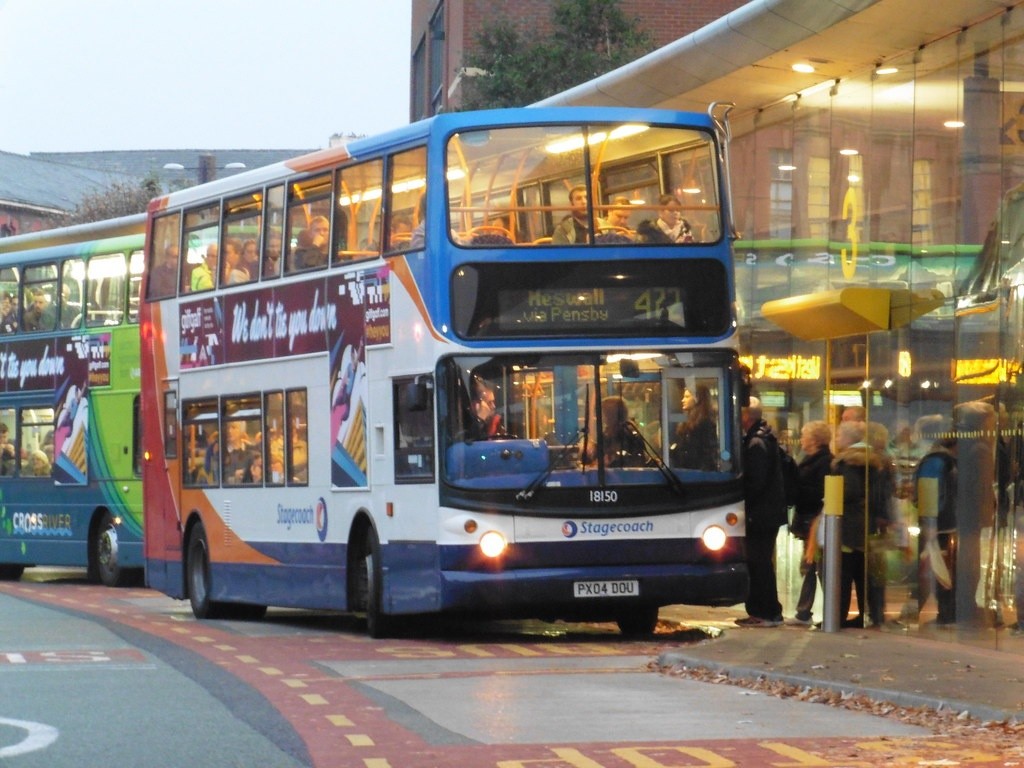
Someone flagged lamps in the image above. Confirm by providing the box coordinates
[752,8,1015,123]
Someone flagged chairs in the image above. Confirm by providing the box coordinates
[466,226,639,246]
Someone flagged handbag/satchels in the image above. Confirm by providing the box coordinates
[779,446,801,506]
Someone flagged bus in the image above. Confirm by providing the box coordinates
[1,211,304,585]
[140,106,748,641]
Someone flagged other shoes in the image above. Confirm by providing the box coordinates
[784,617,814,625]
[815,618,848,629]
[735,614,784,627]
[847,614,877,629]
[891,612,921,628]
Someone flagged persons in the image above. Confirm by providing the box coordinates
[582,396,645,466]
[0,423,54,475]
[359,214,412,259]
[728,398,790,626]
[789,406,901,627]
[447,388,506,440]
[607,196,633,227]
[667,385,717,470]
[554,185,613,244]
[0,283,81,334]
[205,419,307,483]
[913,400,999,628]
[155,215,329,297]
[638,197,693,242]
[411,192,461,248]
